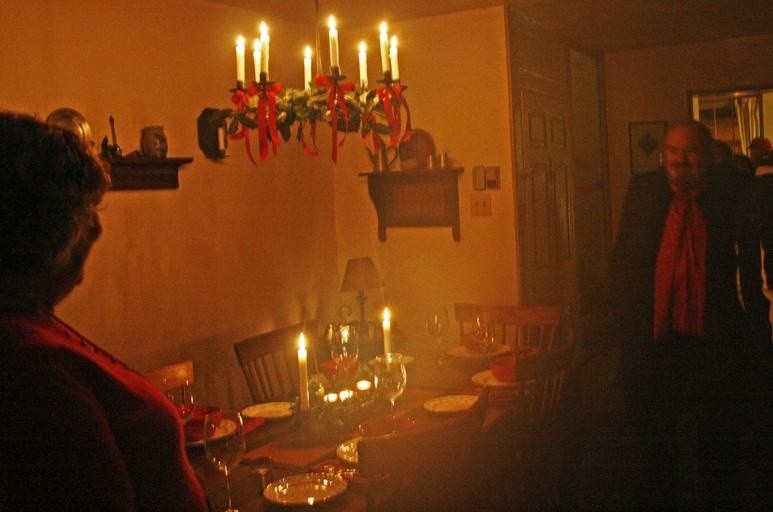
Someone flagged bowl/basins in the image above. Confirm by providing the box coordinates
[464,334,493,354]
[320,359,357,385]
[491,356,531,383]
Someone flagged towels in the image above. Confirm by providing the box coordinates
[176,403,223,442]
[462,335,495,353]
[492,346,542,383]
[321,358,358,384]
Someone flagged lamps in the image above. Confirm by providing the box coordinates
[339,256,383,324]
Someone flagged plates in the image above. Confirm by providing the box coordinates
[242,402,298,421]
[263,473,347,508]
[182,415,236,447]
[448,344,510,362]
[472,369,535,388]
[424,395,480,415]
[368,355,414,368]
[337,437,364,467]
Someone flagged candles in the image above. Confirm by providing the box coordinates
[389,35,399,83]
[381,306,396,377]
[327,14,341,76]
[235,36,246,90]
[302,45,313,92]
[252,21,271,82]
[294,334,312,413]
[357,41,369,91]
[379,20,391,79]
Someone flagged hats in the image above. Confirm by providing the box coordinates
[747,136,772,150]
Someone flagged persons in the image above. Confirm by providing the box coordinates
[592,120,751,511]
[713,137,772,327]
[1,109,208,512]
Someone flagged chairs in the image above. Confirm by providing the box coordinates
[450,300,565,355]
[234,317,321,408]
[139,360,195,405]
[357,418,458,511]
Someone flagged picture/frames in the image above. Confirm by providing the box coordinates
[628,119,666,178]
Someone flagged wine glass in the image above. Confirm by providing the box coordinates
[374,353,406,437]
[160,377,194,429]
[331,326,359,391]
[204,411,245,512]
[426,308,449,362]
